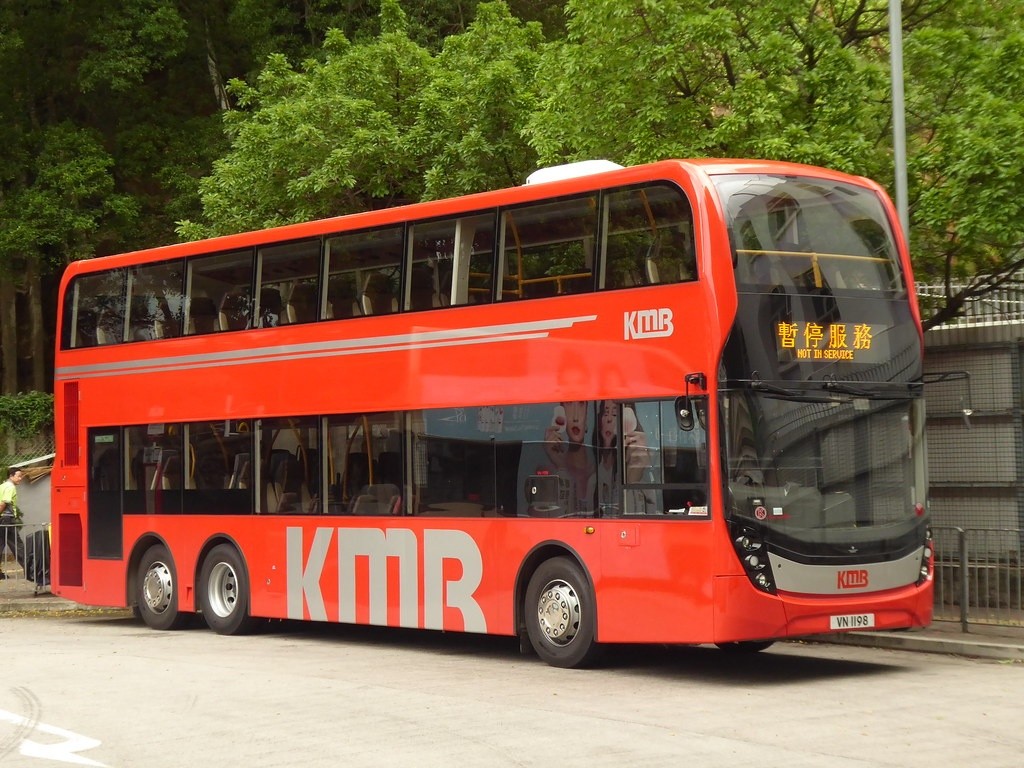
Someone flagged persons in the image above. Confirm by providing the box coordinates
[540,401,657,517]
[267,449,320,515]
[0,468,35,582]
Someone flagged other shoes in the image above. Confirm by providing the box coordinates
[0,572,9,580]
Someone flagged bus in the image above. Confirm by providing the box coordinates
[50,157,976,670]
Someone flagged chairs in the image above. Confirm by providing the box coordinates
[94,431,421,516]
[98,243,808,345]
